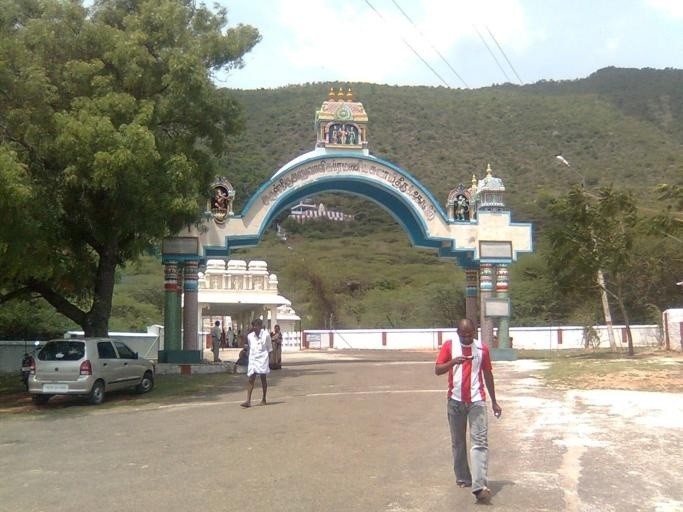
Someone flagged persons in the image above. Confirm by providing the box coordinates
[339,124,347,145]
[212,189,232,208]
[21,352,31,392]
[238,343,249,366]
[226,327,234,347]
[210,320,222,362]
[348,126,355,144]
[332,125,338,143]
[454,195,467,220]
[208,274,264,292]
[239,318,273,407]
[270,325,282,369]
[433,316,502,500]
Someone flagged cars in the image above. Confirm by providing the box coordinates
[27,337,156,405]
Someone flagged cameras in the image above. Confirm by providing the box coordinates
[464,356,474,360]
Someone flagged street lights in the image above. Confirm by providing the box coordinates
[555,154,619,355]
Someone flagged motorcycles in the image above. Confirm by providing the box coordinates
[15,341,43,395]
